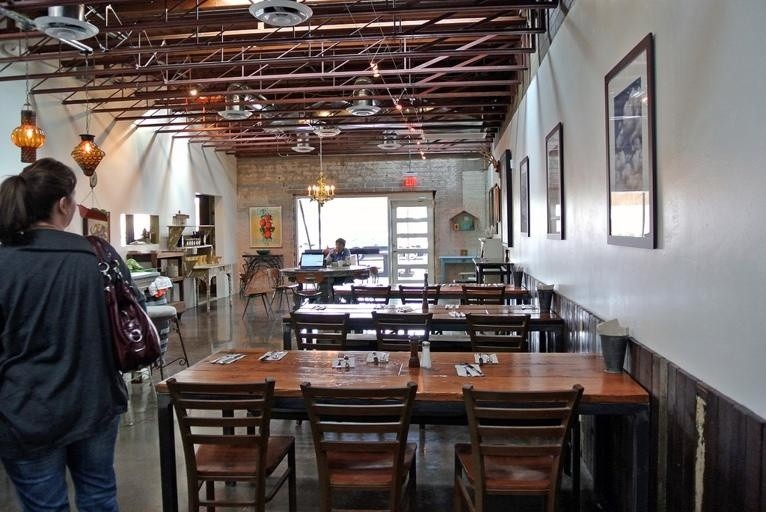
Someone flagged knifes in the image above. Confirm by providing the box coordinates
[259,349,277,362]
[478,350,481,368]
[465,361,485,376]
[221,352,247,365]
[371,352,379,366]
[342,354,349,372]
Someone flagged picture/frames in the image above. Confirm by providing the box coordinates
[500,150,512,248]
[520,155,529,239]
[605,33,656,250]
[84,212,110,244]
[249,206,282,249]
[545,122,564,241]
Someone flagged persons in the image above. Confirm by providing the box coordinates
[0,157,147,512]
[324,238,351,299]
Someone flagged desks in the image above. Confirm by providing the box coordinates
[472,257,514,284]
[438,256,476,283]
[130,271,161,292]
[166,262,233,312]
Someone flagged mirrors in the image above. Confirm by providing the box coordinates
[126,214,159,245]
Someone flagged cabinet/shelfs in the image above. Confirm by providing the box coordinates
[127,251,186,322]
[166,225,217,263]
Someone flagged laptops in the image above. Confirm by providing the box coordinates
[295,253,325,272]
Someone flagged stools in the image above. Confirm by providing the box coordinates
[120,305,189,381]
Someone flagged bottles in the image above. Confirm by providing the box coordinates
[423,272,429,287]
[421,289,428,312]
[184,238,200,247]
[406,334,432,369]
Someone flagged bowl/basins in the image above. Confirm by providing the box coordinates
[256,249,271,256]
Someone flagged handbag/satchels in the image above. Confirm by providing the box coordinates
[102,279,160,373]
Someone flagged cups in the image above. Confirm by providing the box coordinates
[599,333,629,374]
[512,271,523,289]
[537,288,554,313]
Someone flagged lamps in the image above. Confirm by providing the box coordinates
[72,65,106,177]
[12,39,45,163]
[307,136,336,206]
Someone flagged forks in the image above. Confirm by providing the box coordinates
[335,352,344,370]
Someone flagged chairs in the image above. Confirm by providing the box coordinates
[166,374,298,512]
[281,285,566,354]
[300,380,419,511]
[241,268,276,318]
[271,268,299,311]
[452,381,584,512]
